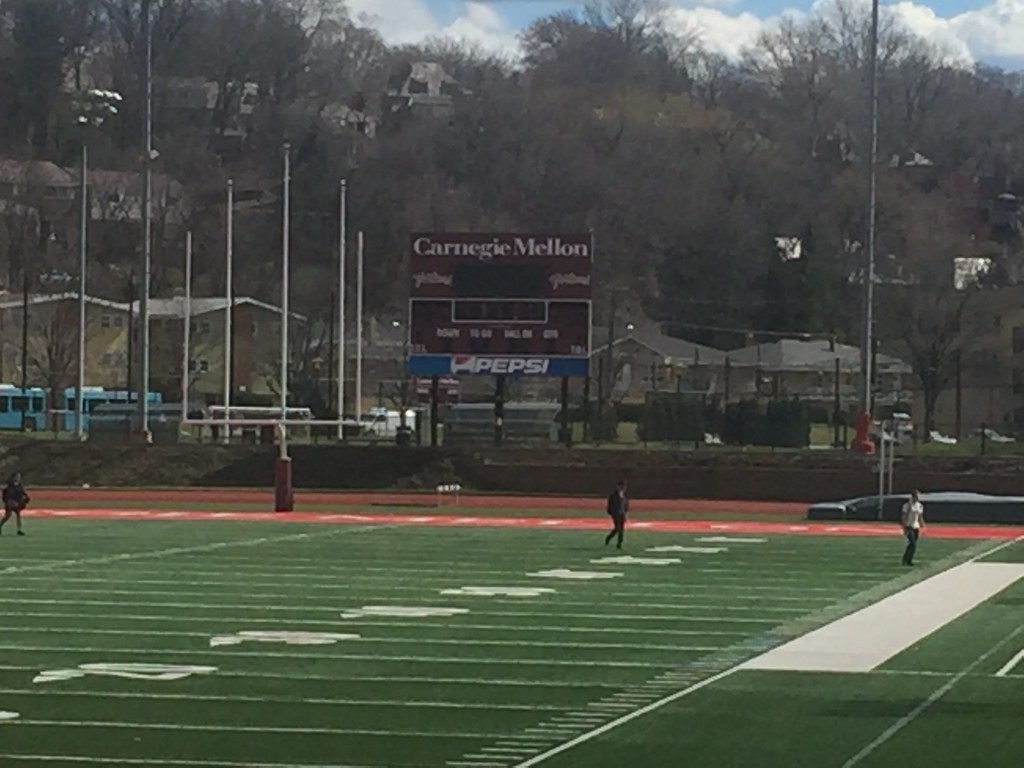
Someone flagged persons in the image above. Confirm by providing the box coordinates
[605,480,629,548]
[0,471,30,536]
[901,490,924,567]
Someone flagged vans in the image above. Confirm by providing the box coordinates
[0,388,47,433]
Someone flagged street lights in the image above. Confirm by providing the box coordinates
[62,85,121,436]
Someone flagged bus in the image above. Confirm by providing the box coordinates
[64,388,162,433]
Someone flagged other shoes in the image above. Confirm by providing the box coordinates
[617,544,622,549]
[17,530,24,536]
[902,559,914,566]
[606,537,610,544]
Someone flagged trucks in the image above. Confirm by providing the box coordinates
[358,410,416,436]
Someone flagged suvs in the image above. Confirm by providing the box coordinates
[964,428,1016,445]
